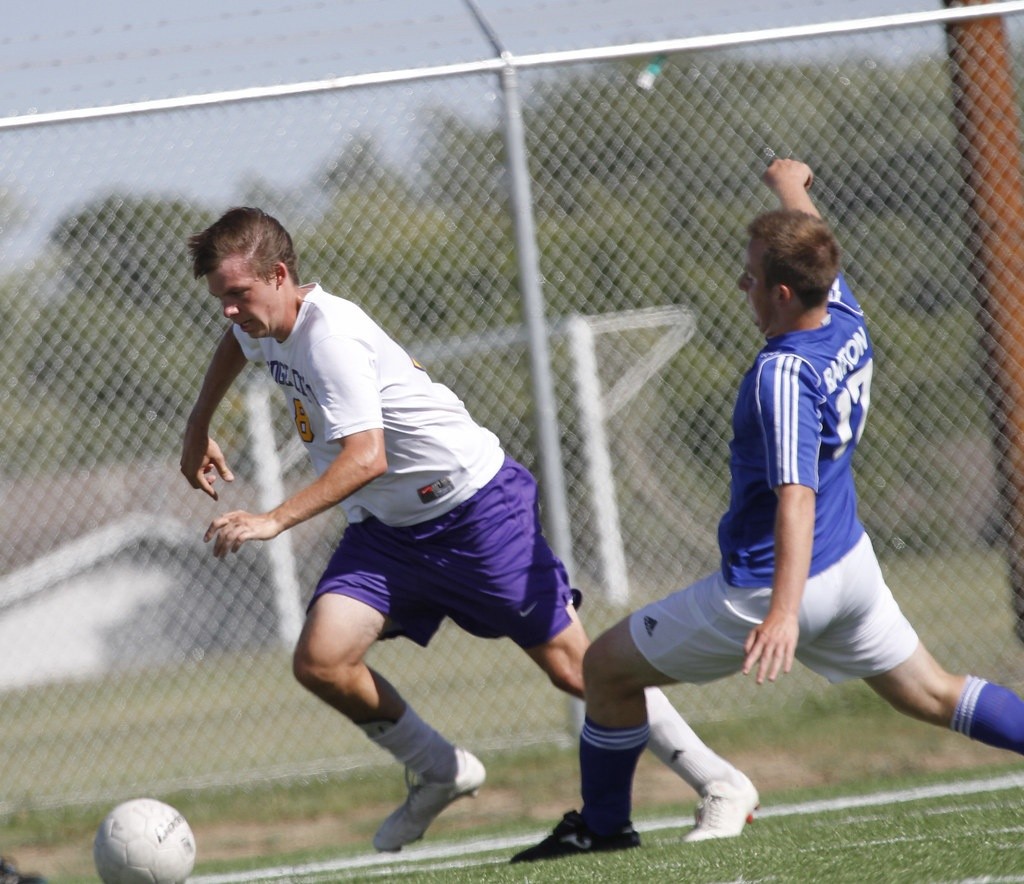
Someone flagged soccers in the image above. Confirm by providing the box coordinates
[94,797,198,884]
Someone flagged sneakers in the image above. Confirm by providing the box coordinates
[511,809,639,863]
[373,747,485,851]
[684,769,759,842]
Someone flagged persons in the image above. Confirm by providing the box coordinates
[509,158,1024,863]
[180,207,760,850]
